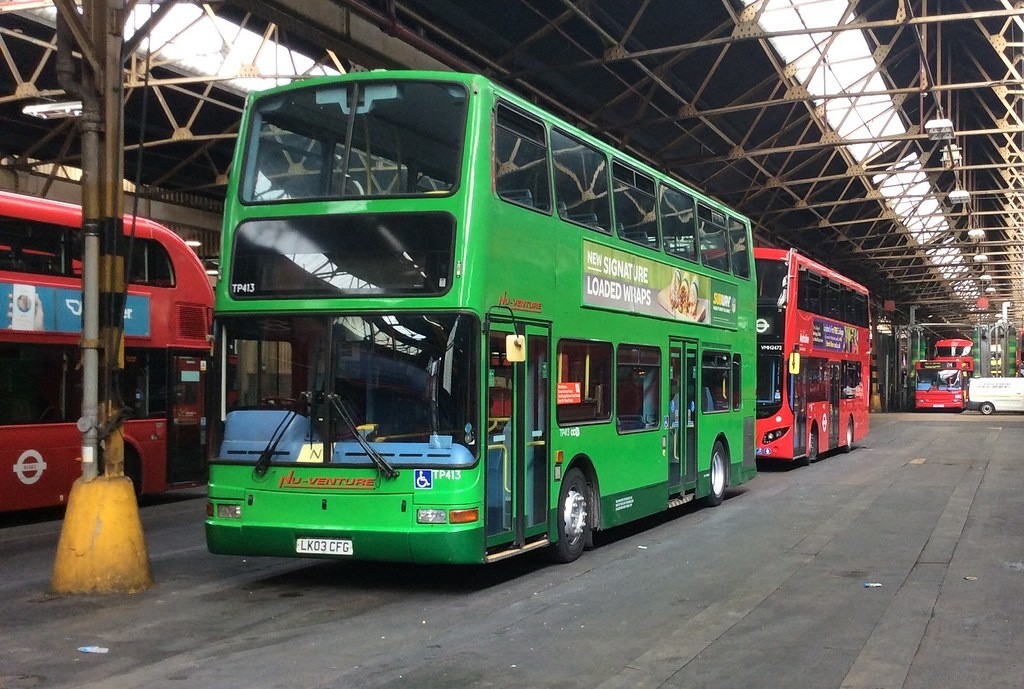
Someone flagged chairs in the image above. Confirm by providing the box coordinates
[498,188,534,207]
[702,386,715,412]
[27,255,53,275]
[668,247,690,259]
[536,202,568,217]
[567,213,598,226]
[624,231,650,244]
[649,239,670,253]
[617,375,655,430]
[601,222,626,237]
[690,254,708,264]
[709,257,725,270]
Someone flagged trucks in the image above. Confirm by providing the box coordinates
[990,344,1003,375]
[932,338,975,373]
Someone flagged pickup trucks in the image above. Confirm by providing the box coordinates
[965,377,1024,415]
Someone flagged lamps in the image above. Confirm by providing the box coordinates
[924,109,956,141]
[939,144,963,169]
[967,228,986,239]
[973,254,988,262]
[985,288,996,293]
[949,179,971,204]
[22,100,82,120]
[979,274,992,281]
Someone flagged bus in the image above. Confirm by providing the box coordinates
[201,69,757,568]
[0,191,215,517]
[910,359,971,413]
[753,247,873,464]
